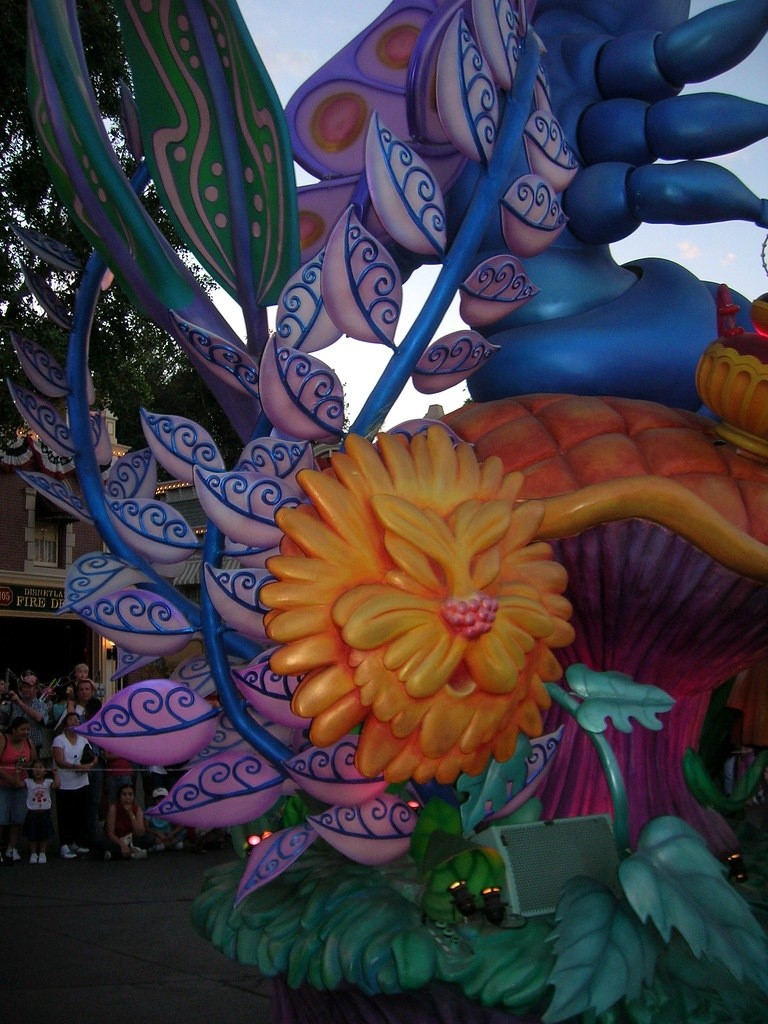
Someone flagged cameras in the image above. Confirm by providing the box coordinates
[1,692,13,701]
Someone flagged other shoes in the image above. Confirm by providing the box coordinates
[60,846,77,859]
[198,843,208,854]
[218,837,225,844]
[72,843,90,853]
[177,842,183,849]
[131,848,148,859]
[104,851,111,861]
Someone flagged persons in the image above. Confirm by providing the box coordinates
[105,784,147,862]
[0,663,134,864]
[143,788,184,854]
[195,827,232,849]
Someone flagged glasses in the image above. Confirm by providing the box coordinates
[152,796,165,803]
[66,718,80,723]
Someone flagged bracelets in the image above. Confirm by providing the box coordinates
[90,763,94,768]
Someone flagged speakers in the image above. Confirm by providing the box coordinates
[472,813,619,918]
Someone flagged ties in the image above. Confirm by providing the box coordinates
[20,681,36,689]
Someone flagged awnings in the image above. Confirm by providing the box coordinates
[173,557,244,586]
[35,490,80,526]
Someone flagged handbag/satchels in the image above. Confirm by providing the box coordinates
[78,744,94,765]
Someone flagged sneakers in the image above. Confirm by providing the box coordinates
[38,852,47,864]
[5,849,13,858]
[12,848,21,861]
[29,853,38,864]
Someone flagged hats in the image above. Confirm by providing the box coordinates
[152,787,169,798]
